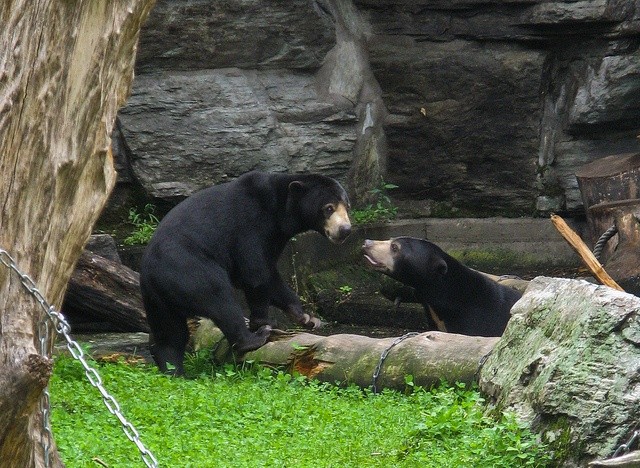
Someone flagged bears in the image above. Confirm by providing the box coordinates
[137,167,354,375]
[356,235,527,338]
